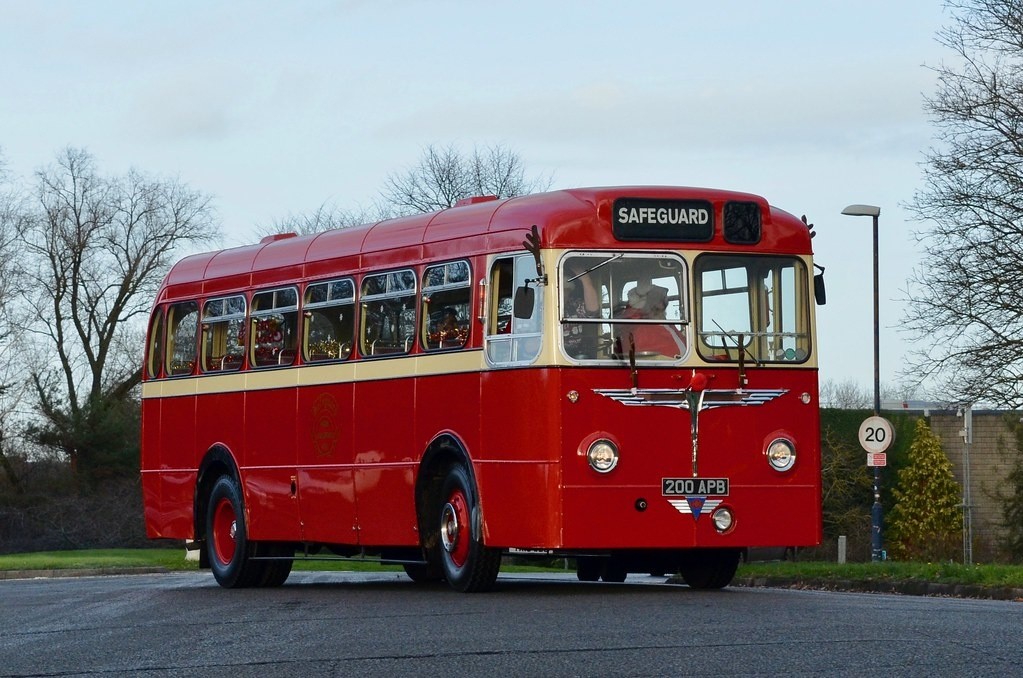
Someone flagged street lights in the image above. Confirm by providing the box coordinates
[841,204,889,560]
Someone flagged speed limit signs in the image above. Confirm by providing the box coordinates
[858,415,891,452]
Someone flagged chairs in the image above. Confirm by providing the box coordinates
[220,354,243,371]
[439,336,459,348]
[255,348,277,366]
[277,348,296,364]
[370,338,413,355]
[338,342,396,358]
[308,349,333,361]
[171,360,193,375]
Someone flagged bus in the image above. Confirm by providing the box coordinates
[140,184,830,592]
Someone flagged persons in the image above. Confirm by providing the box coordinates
[431,308,460,348]
[562,258,601,359]
[614,262,686,359]
